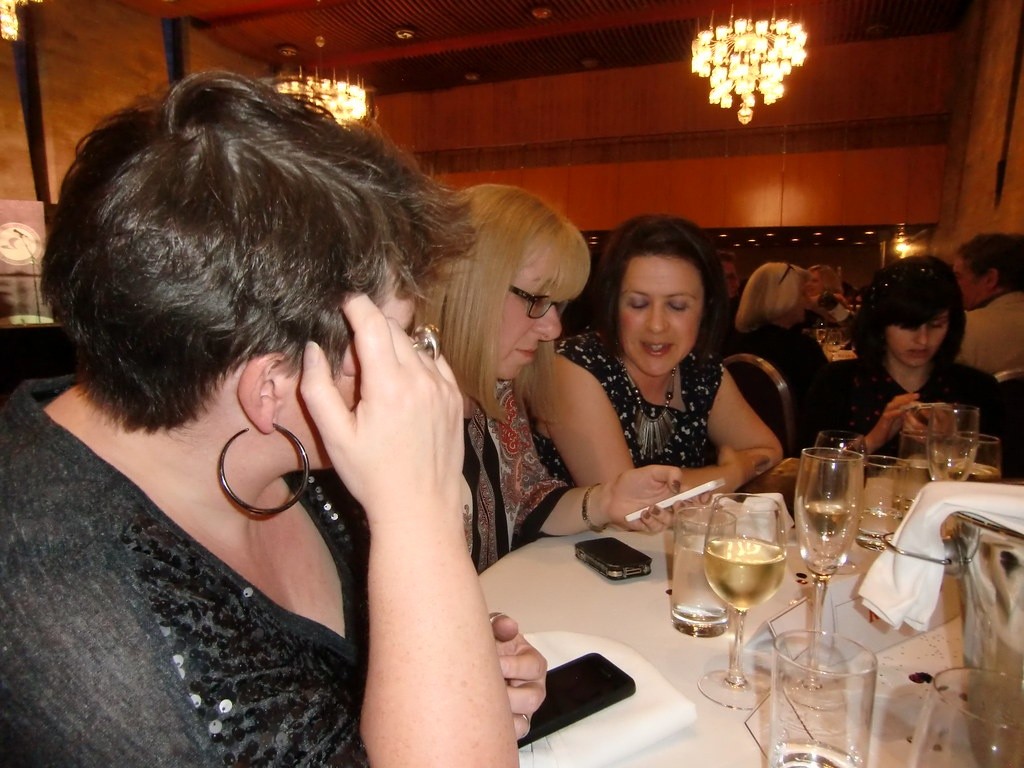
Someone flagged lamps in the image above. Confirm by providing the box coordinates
[689,0,807,127]
[273,35,366,125]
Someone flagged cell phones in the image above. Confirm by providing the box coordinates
[515,652,637,750]
[626,478,725,522]
[912,402,946,423]
[576,537,651,579]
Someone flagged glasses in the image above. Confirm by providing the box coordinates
[870,262,938,310]
[506,284,568,320]
[778,262,796,289]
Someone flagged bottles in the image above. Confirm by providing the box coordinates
[818,291,855,329]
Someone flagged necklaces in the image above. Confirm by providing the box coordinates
[618,357,677,460]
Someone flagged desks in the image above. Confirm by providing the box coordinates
[477,481,1024,768]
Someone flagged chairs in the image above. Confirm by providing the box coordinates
[724,351,793,458]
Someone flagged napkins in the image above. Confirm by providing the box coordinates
[711,492,795,543]
[519,630,696,767]
[858,480,1024,629]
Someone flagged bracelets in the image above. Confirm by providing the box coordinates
[582,483,608,532]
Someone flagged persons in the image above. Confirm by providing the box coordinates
[834,254,1008,477]
[735,261,834,458]
[528,213,783,494]
[0,68,548,768]
[952,231,1024,375]
[406,183,714,576]
[822,290,856,326]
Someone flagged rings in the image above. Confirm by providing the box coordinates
[490,612,503,621]
[412,322,441,360]
[523,714,530,737]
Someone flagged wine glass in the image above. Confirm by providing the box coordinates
[698,493,787,711]
[824,331,842,361]
[784,447,863,711]
[814,430,867,575]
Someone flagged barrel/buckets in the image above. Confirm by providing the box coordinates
[880,511,1024,767]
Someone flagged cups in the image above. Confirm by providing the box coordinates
[910,667,1024,768]
[890,403,1001,517]
[855,454,911,553]
[670,508,736,638]
[766,630,878,767]
